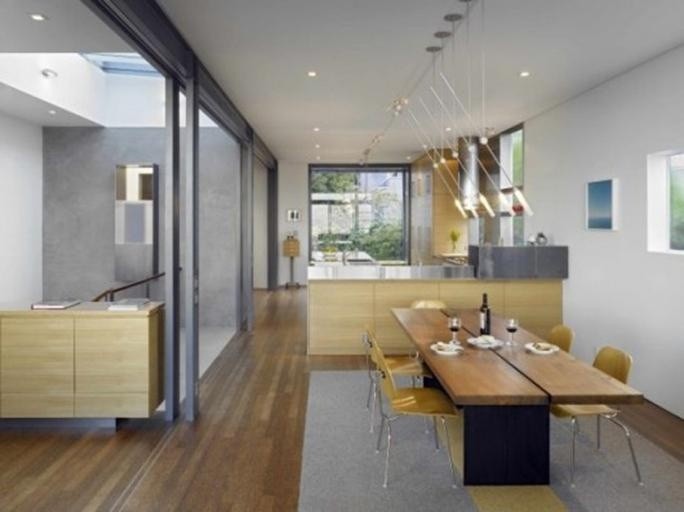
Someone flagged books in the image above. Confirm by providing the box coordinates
[31,300,81,311]
[106,297,151,310]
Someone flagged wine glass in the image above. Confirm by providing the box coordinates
[503,315,521,346]
[447,316,463,345]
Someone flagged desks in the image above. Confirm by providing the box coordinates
[317,249,376,264]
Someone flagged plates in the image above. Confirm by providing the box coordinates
[467,336,503,350]
[524,342,561,354]
[430,344,466,356]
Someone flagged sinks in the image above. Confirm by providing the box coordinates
[347,258,372,263]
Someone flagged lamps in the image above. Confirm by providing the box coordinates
[282,235,300,289]
[394,3,539,222]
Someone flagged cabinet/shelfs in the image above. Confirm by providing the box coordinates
[0,302,165,432]
[408,147,468,265]
[304,262,564,357]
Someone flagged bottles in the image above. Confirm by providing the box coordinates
[480,293,491,334]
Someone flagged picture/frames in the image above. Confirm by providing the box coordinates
[583,177,619,232]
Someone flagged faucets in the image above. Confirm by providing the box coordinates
[342,246,359,261]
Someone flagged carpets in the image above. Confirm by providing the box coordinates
[295,366,684,511]
